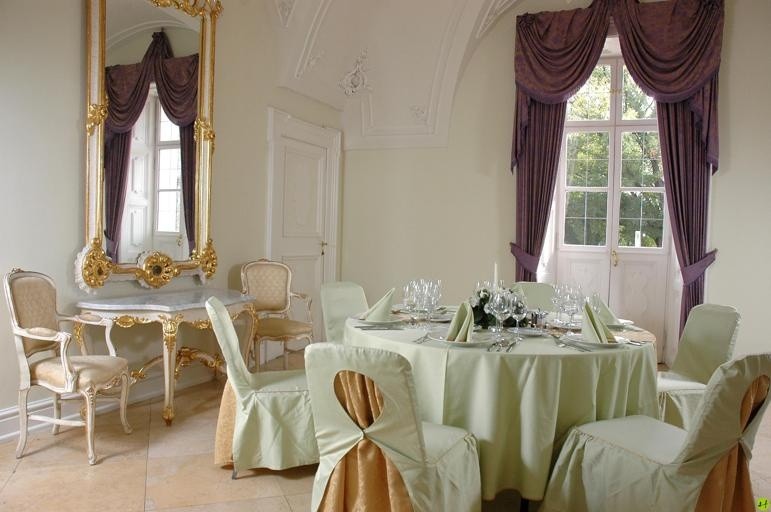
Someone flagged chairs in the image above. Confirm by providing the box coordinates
[544,353,771,511]
[658,302,743,431]
[204,294,321,479]
[506,279,556,309]
[4,267,132,467]
[237,260,318,370]
[303,342,481,511]
[320,280,371,344]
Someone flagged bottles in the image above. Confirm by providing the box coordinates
[530,308,547,328]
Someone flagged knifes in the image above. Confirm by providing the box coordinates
[488,338,522,353]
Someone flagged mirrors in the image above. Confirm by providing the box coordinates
[75,1,221,288]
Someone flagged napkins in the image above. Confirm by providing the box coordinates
[443,301,477,342]
[576,299,617,345]
[599,300,625,326]
[357,286,396,321]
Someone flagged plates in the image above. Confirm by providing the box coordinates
[425,313,455,323]
[560,319,635,349]
[507,328,547,337]
[425,332,497,347]
[348,312,407,325]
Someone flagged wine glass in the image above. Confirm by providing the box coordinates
[551,283,585,346]
[402,276,443,337]
[476,280,528,355]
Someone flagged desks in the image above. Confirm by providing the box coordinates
[343,301,661,503]
[76,286,256,424]
